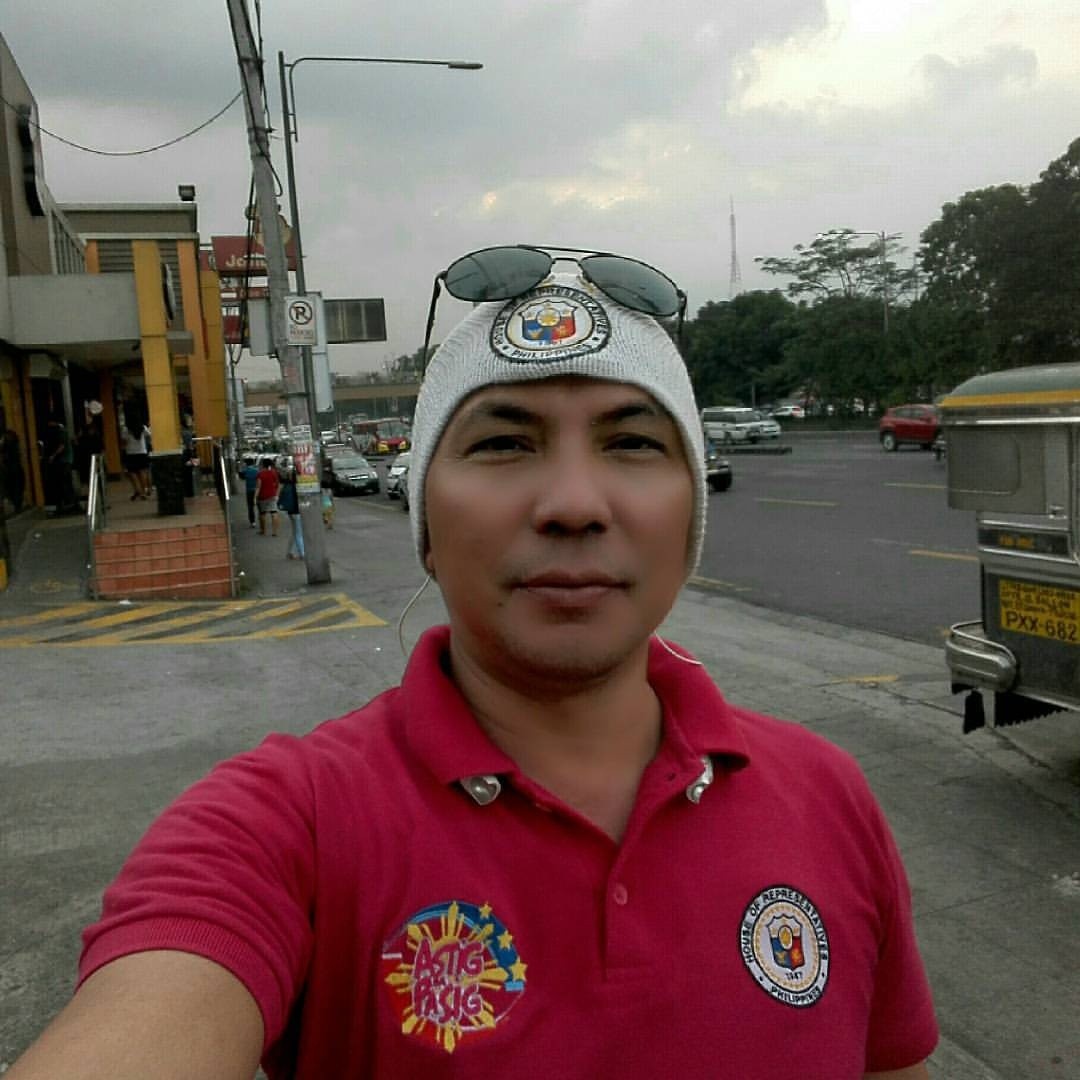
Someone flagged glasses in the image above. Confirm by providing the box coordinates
[421,246,689,376]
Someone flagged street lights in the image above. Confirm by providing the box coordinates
[278,50,484,524]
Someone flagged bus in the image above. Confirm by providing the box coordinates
[352,416,412,456]
[700,406,762,446]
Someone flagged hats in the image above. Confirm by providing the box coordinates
[407,272,712,574]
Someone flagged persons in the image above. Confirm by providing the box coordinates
[253,458,281,536]
[285,468,305,559]
[0,243,940,1080]
[237,456,261,527]
[119,408,153,500]
[320,480,336,527]
[37,399,105,517]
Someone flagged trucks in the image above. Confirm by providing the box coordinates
[940,360,1080,735]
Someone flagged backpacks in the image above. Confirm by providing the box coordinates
[276,483,296,513]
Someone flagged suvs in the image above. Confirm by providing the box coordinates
[879,404,940,452]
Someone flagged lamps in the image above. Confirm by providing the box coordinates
[179,185,196,201]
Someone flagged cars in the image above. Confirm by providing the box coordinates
[232,422,356,491]
[324,455,380,497]
[386,452,411,498]
[754,410,781,439]
[827,397,877,418]
[703,433,734,492]
[397,466,411,512]
[765,406,804,418]
